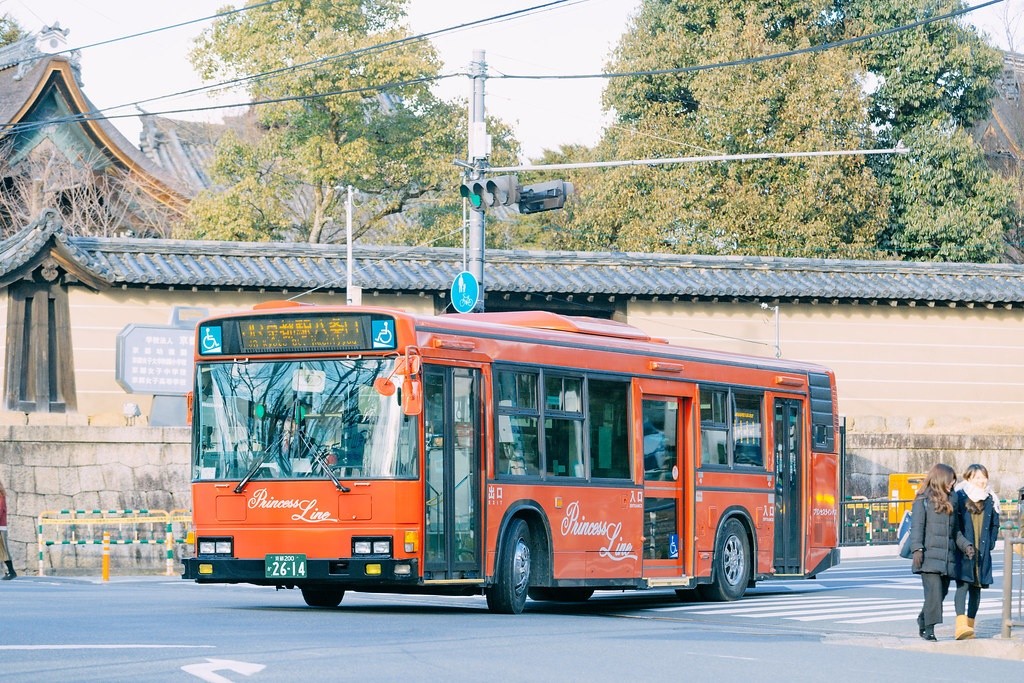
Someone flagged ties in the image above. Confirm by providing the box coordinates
[283,440,288,451]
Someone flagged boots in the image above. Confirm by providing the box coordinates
[955,614,975,640]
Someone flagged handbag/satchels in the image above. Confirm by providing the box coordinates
[897,494,928,559]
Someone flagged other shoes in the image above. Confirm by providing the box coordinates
[924,624,937,642]
[917,616,926,637]
[3,571,17,580]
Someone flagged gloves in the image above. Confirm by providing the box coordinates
[965,546,975,561]
[913,550,923,569]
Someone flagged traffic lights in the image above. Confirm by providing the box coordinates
[461,177,519,212]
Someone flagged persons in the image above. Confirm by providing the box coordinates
[910,463,974,642]
[0,483,18,580]
[955,464,1001,640]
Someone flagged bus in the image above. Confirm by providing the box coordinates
[178,301,840,616]
